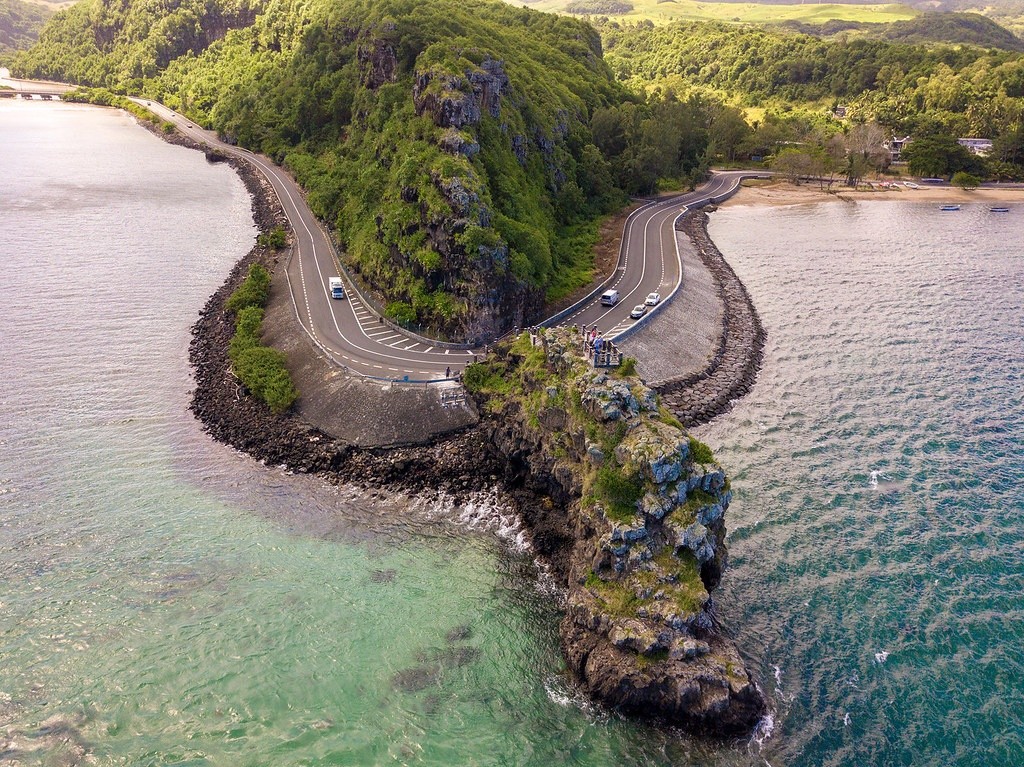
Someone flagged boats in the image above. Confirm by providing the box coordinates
[989,207,1009,212]
[938,204,961,211]
[903,181,919,189]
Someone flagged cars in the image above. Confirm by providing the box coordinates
[643,292,660,306]
[630,304,648,319]
[187,123,193,128]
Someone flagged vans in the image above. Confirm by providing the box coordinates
[600,289,619,307]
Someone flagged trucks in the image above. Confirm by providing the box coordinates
[328,276,345,300]
